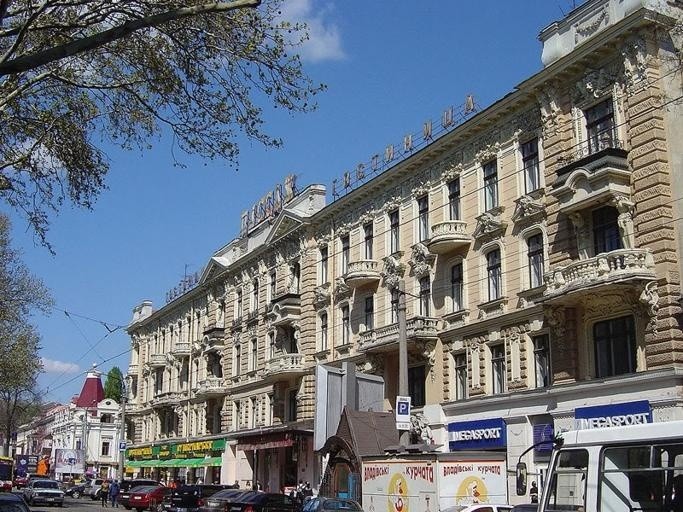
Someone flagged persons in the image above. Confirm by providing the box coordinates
[157,476,183,488]
[289,479,312,506]
[195,477,202,484]
[254,479,261,490]
[107,479,120,507]
[529,481,538,502]
[99,478,108,507]
[244,480,250,489]
[232,479,240,488]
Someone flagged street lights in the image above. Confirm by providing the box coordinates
[83,398,95,473]
[69,421,77,479]
[84,369,128,487]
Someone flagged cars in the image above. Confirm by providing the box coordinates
[0,472,65,512]
[222,491,303,512]
[63,473,238,512]
[508,503,587,511]
[197,489,254,512]
[438,503,515,512]
[297,496,364,512]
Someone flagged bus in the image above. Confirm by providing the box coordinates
[0,455,13,491]
[511,418,683,511]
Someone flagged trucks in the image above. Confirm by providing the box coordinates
[343,449,509,512]
